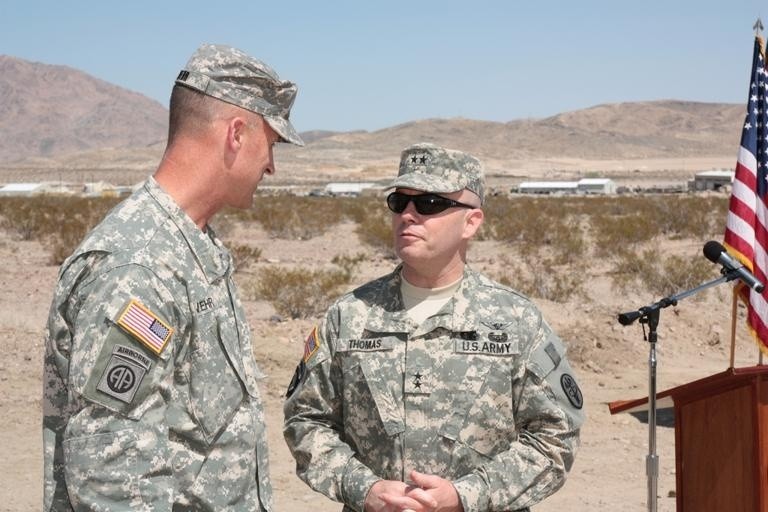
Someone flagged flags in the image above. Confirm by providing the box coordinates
[722,34,768,358]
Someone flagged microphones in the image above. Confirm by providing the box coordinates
[703,240,766,293]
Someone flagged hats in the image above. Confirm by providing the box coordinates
[383,142,484,202]
[175,43,305,147]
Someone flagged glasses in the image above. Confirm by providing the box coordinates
[387,192,475,215]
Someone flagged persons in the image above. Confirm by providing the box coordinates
[42,43,305,512]
[284,143,584,512]
